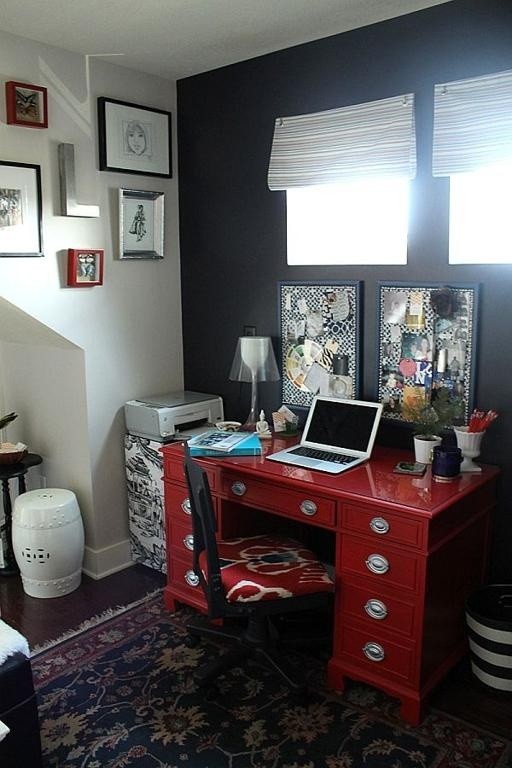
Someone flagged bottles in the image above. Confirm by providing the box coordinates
[434,348,464,397]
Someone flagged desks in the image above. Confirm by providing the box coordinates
[0,450,44,582]
[157,421,504,727]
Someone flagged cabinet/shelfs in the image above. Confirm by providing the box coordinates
[120,432,172,580]
[0,612,44,766]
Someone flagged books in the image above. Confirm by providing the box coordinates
[180,429,262,458]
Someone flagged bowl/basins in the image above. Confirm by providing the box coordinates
[215,421,243,433]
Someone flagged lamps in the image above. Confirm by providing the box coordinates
[228,335,280,432]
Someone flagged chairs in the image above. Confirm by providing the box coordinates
[179,457,336,706]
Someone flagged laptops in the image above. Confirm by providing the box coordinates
[265,396,384,474]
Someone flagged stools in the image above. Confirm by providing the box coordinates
[9,486,88,601]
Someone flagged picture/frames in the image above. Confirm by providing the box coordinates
[373,277,482,436]
[0,159,46,261]
[95,92,174,180]
[4,77,48,129]
[115,187,167,264]
[274,278,365,415]
[65,247,106,290]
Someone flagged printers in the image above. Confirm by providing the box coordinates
[124,390,225,443]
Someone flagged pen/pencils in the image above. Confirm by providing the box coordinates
[467,408,499,433]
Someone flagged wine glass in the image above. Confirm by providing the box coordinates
[453,426,483,472]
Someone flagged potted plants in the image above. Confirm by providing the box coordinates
[400,385,465,466]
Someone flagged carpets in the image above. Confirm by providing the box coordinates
[31,588,511,767]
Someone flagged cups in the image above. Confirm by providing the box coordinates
[429,445,462,483]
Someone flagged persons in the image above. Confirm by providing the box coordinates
[385,371,397,389]
[411,341,421,360]
[450,356,460,376]
[253,410,272,435]
[418,337,433,362]
